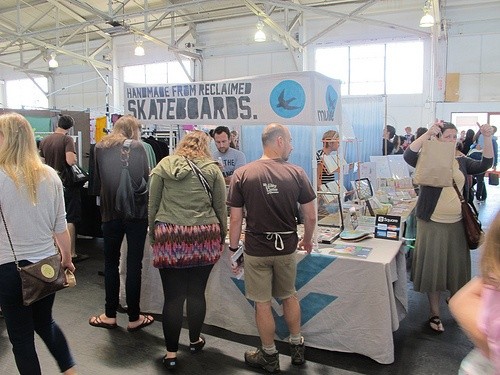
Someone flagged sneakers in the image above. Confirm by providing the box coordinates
[288,336,305,365]
[244,347,280,372]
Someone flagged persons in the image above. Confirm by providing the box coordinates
[0,114,500,375]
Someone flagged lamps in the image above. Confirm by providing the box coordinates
[254,20,266,42]
[134,33,145,56]
[48,51,58,68]
[419,0,435,28]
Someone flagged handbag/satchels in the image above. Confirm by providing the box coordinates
[461,200,485,251]
[18,253,69,306]
[116,138,148,221]
[63,161,89,184]
[414,134,456,187]
[488,173,499,185]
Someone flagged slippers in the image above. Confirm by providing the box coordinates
[88,315,118,329]
[127,315,155,330]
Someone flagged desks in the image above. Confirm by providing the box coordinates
[117,192,419,366]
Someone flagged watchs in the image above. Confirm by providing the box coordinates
[229,245,240,252]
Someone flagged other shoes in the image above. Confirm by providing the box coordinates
[162,353,179,370]
[428,317,445,334]
[190,336,206,353]
